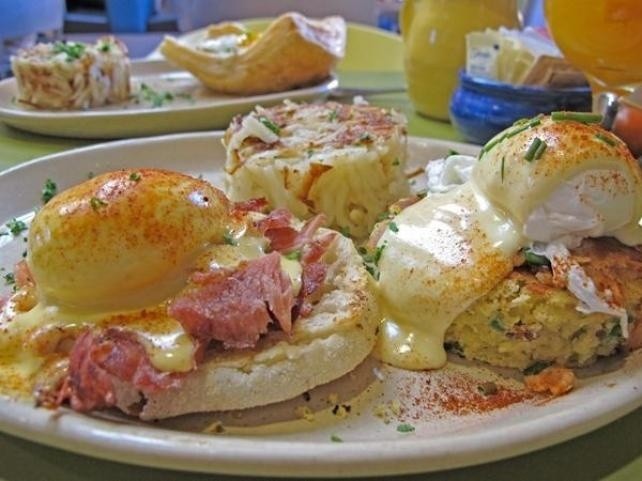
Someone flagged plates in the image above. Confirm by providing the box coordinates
[0,128,642,476]
[1,57,341,141]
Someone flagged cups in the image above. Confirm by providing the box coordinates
[400,1,528,123]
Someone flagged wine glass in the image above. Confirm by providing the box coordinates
[542,0,642,133]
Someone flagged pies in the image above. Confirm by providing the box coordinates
[114,226,382,421]
